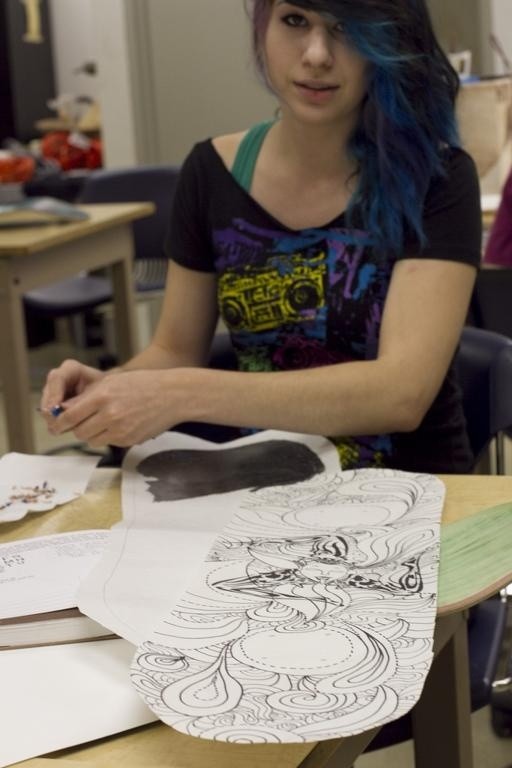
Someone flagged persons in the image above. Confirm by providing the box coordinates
[33,0,488,749]
[483,166,511,270]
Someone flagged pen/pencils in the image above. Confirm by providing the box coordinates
[34,402,64,417]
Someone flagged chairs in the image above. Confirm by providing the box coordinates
[97,324,512,754]
[18,164,181,364]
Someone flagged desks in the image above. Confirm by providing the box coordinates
[0,199,158,450]
[0,465,512,768]
